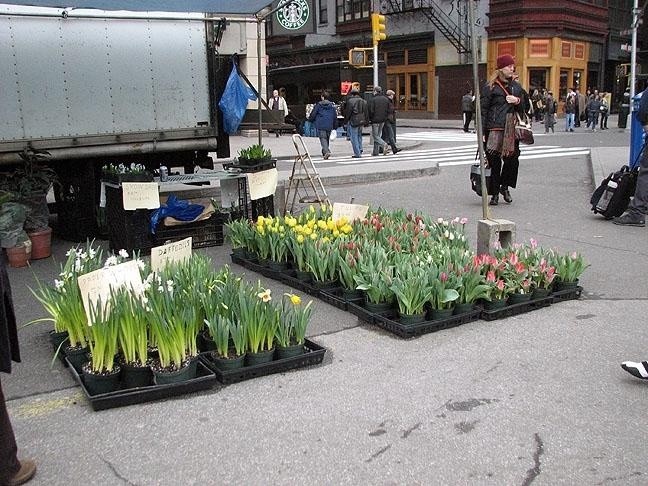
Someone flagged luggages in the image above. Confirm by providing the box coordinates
[590,143,645,220]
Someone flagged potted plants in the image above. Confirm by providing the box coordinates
[0,142,62,267]
[237,144,272,166]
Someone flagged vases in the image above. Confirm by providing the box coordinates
[398,313,427,326]
[80,363,120,395]
[105,173,152,186]
[155,365,190,385]
[121,365,154,389]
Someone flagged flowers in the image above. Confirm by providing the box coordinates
[104,162,146,174]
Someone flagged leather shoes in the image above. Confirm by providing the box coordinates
[490,195,498,205]
[499,188,512,202]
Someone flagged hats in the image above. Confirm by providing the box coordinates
[495,54,514,70]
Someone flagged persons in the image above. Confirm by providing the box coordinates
[461,87,475,133]
[309,91,338,160]
[621,359,648,378]
[480,54,530,206]
[612,88,647,227]
[269,89,289,138]
[513,74,609,135]
[1,251,38,485]
[340,86,402,158]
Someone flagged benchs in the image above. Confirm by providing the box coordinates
[239,109,296,137]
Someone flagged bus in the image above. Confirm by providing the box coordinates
[267,59,387,131]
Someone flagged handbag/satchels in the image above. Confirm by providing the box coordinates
[350,113,366,127]
[471,164,492,195]
[514,121,534,144]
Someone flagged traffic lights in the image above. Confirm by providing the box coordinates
[370,12,386,45]
[347,48,367,65]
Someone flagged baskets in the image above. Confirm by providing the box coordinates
[150,216,224,249]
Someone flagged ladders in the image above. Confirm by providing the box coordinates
[281,134,333,220]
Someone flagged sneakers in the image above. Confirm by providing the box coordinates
[613,209,644,227]
[352,149,363,159]
[620,361,648,379]
[0,459,36,485]
[372,141,401,156]
[322,148,331,159]
